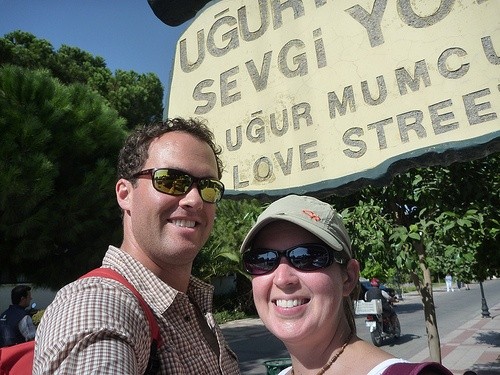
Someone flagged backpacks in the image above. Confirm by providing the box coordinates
[0,268,164,375]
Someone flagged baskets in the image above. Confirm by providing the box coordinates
[353,299,383,315]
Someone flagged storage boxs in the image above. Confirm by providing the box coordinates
[354,299,382,314]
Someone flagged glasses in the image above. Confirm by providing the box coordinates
[130,168,225,204]
[242,243,344,275]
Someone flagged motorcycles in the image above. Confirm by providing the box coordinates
[354,296,401,347]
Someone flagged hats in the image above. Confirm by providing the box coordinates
[239,195,351,260]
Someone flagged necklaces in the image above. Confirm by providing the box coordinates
[291,328,356,375]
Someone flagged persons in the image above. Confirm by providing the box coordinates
[31,116,242,375]
[364,277,397,335]
[445,273,470,293]
[0,285,36,348]
[249,194,416,375]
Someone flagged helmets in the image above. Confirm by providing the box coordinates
[371,278,379,286]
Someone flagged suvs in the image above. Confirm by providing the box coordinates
[354,277,395,301]
